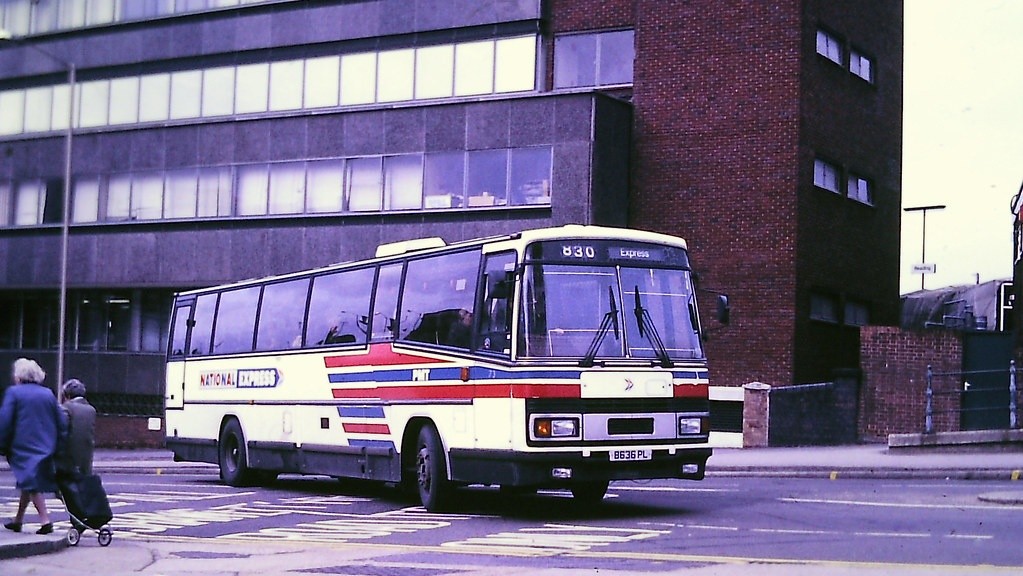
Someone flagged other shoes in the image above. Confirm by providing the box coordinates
[4,522,22,532]
[36,521,53,534]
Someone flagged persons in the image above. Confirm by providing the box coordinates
[0,358,71,535]
[446,305,474,348]
[59,379,97,496]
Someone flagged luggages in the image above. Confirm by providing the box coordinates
[55,469,114,547]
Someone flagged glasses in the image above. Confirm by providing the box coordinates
[468,313,473,318]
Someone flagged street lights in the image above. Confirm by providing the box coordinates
[1,29,75,405]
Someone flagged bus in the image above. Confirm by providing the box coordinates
[165,224,731,515]
